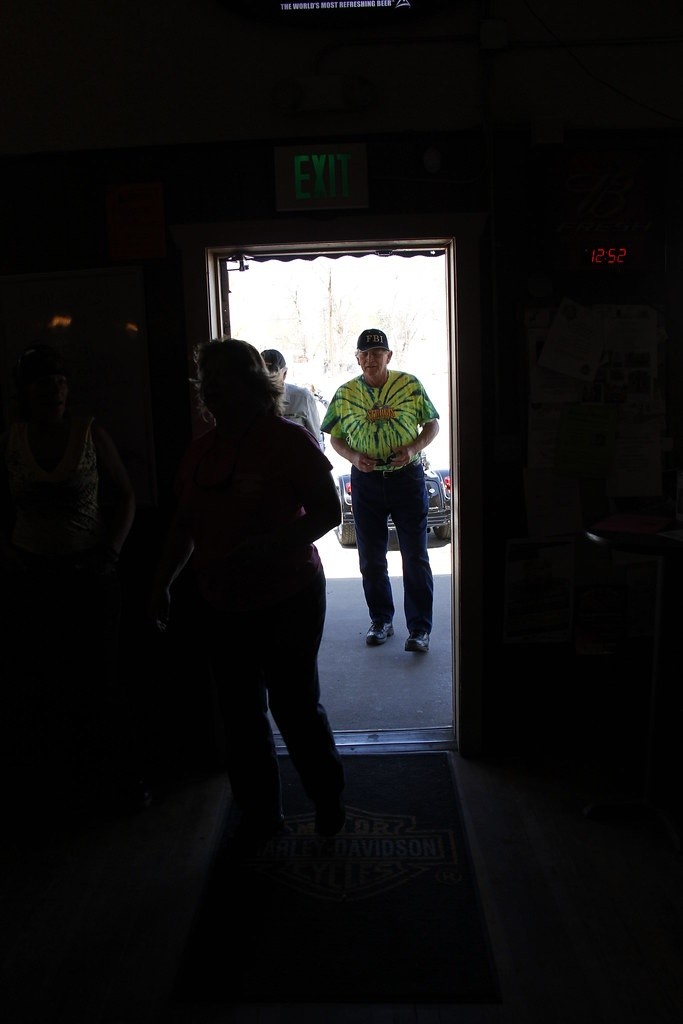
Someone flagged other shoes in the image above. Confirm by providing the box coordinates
[226,810,286,867]
[314,793,346,838]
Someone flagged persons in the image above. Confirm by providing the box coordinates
[150,341,346,853]
[321,329,438,652]
[260,349,315,438]
[0,338,136,821]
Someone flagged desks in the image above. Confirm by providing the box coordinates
[581,511,683,855]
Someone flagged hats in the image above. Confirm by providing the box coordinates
[357,329,390,352]
[261,349,286,373]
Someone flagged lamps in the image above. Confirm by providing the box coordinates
[396,140,445,176]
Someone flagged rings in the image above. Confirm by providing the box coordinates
[367,463,370,467]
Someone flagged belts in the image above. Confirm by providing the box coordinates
[379,457,421,479]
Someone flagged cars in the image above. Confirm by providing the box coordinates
[334,450,450,545]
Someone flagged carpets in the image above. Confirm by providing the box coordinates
[169,753,504,1007]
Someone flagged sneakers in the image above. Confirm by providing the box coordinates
[405,631,430,651]
[366,622,394,645]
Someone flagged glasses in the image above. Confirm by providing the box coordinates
[366,442,397,466]
